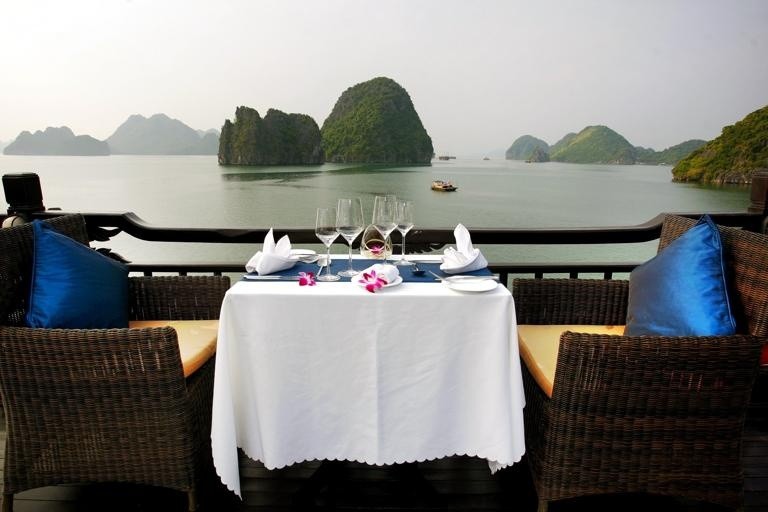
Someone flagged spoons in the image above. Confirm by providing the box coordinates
[411,263,425,275]
[315,257,332,278]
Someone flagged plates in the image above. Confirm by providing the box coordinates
[291,248,317,260]
[441,275,500,293]
[351,270,404,288]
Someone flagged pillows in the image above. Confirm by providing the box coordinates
[27,218,131,330]
[623,213,738,336]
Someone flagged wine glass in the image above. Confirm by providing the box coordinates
[336,197,365,277]
[315,206,341,282]
[371,194,398,267]
[392,200,416,267]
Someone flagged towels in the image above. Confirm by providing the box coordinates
[245,226,299,276]
[437,223,489,275]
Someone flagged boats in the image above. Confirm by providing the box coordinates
[431,179,457,192]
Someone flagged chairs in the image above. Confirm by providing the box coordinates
[1,213,231,512]
[510,213,768,512]
